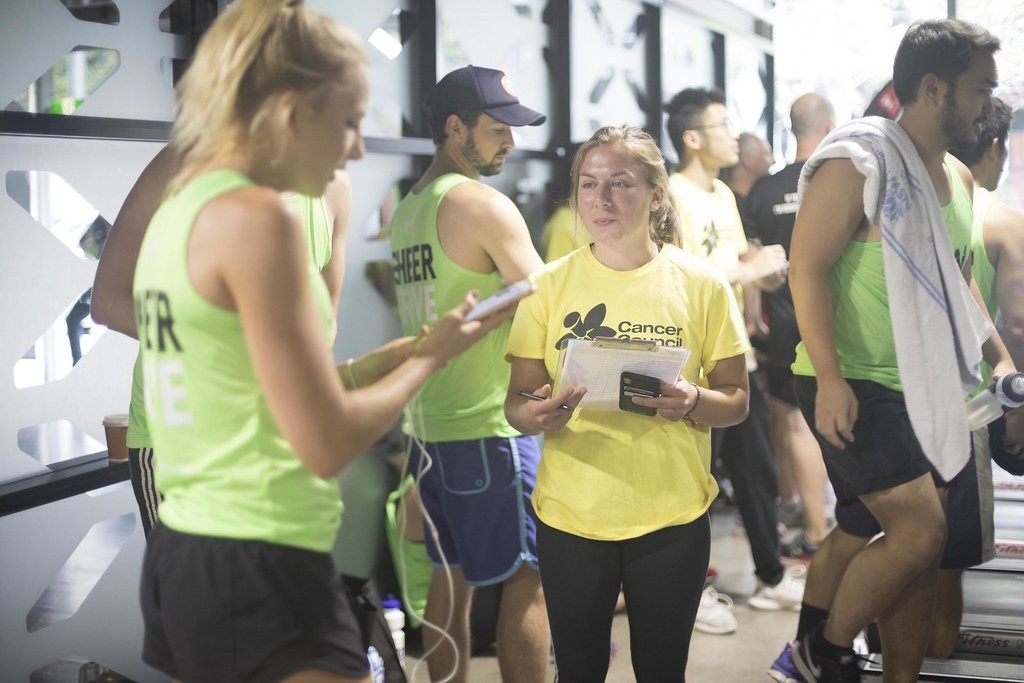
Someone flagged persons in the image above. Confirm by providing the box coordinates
[726,130,836,536]
[500,124,751,683]
[738,89,849,557]
[538,137,597,263]
[765,17,1024,683]
[87,0,519,682]
[656,88,809,637]
[388,63,553,682]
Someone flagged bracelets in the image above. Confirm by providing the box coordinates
[347,358,359,391]
[682,383,701,426]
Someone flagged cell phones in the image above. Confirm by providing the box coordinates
[618,371,662,416]
[467,278,537,324]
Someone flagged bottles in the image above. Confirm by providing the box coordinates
[381,593,406,673]
[996,372,1024,407]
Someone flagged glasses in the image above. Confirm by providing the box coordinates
[692,118,735,132]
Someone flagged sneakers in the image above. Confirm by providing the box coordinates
[778,535,823,569]
[767,639,808,682]
[790,621,864,682]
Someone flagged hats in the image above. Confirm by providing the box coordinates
[430,65,547,127]
